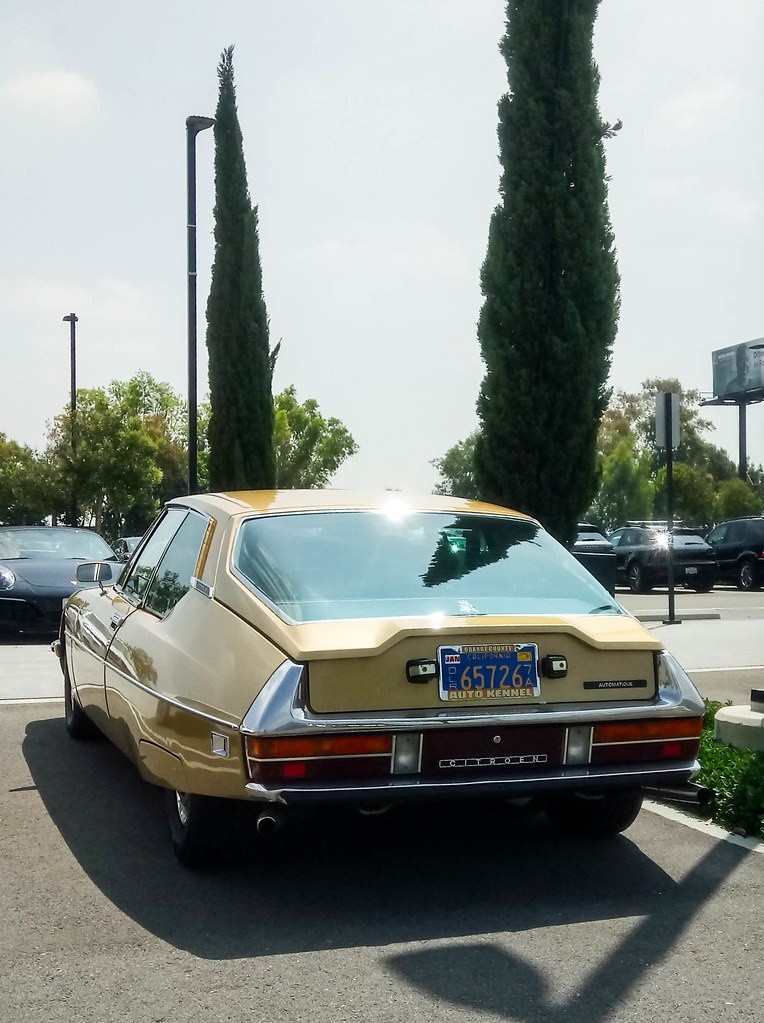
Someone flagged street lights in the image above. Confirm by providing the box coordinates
[62,312,79,527]
[184,113,216,496]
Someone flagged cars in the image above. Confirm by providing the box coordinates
[569,520,618,598]
[48,486,708,861]
[109,535,144,563]
[0,525,129,634]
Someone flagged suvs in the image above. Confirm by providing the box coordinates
[702,514,764,591]
[606,517,721,595]
